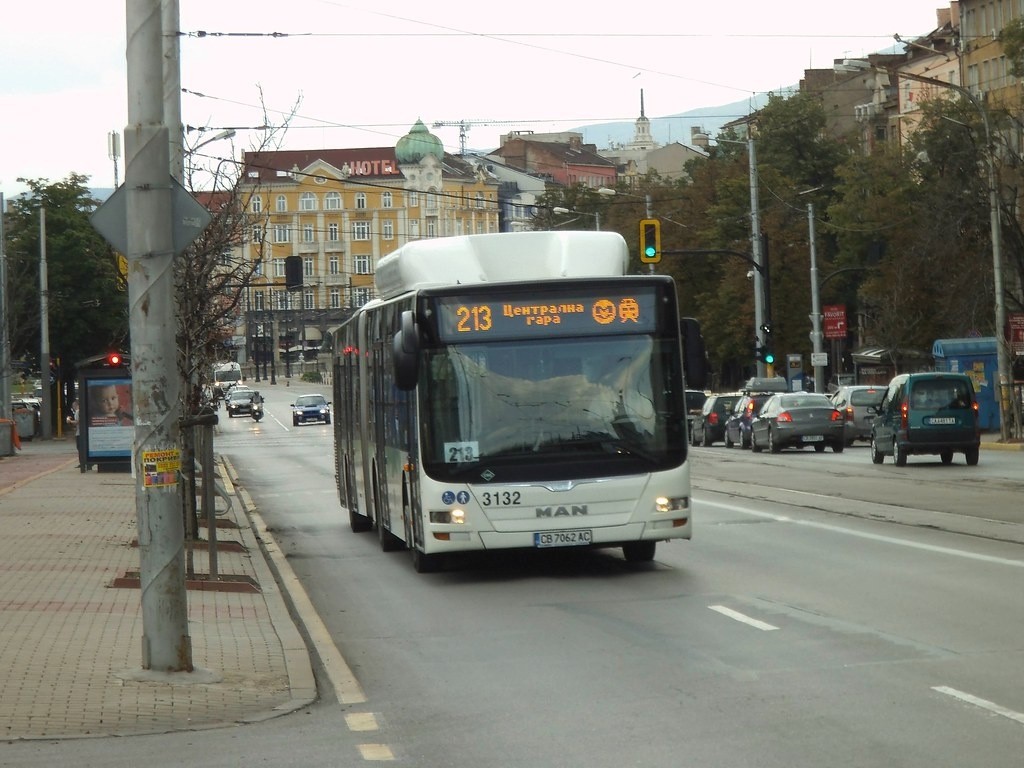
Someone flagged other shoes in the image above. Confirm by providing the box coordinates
[76,465,81,468]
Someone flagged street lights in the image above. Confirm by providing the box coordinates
[692,130,767,377]
[553,207,600,231]
[598,187,656,274]
[834,60,1013,444]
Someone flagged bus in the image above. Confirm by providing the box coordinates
[319,230,710,575]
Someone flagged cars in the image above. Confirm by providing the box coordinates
[224,385,250,411]
[750,391,846,453]
[685,387,784,450]
[227,390,257,418]
[290,394,332,426]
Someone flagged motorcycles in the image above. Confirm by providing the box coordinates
[249,397,265,422]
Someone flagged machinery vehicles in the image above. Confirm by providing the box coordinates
[211,361,246,399]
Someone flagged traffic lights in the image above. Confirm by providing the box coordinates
[639,219,661,263]
[763,352,775,365]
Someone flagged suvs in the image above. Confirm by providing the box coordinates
[827,384,890,447]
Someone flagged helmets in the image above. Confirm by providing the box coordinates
[254,390,259,396]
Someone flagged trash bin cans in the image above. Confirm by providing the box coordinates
[0,419,16,456]
[12,409,34,442]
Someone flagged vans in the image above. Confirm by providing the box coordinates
[869,371,981,467]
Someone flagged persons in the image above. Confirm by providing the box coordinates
[67,398,80,467]
[90,384,134,426]
[588,371,657,438]
[248,392,264,404]
